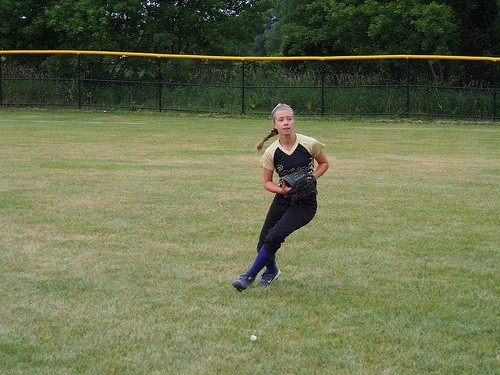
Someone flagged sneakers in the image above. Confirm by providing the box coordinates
[231,273,254,292]
[260,268,281,285]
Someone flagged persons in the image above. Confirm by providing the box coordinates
[231,103,328,293]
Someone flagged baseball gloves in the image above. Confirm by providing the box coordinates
[279,172,317,201]
